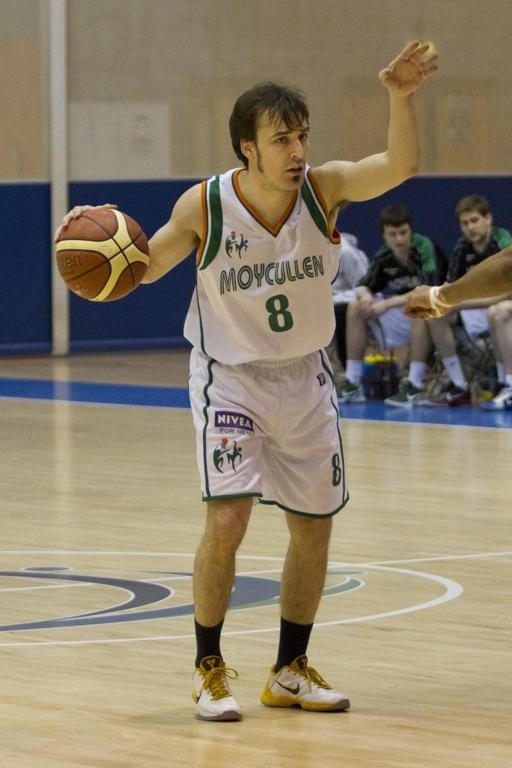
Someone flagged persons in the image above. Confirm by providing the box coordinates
[333,194,511,411]
[53,42,439,721]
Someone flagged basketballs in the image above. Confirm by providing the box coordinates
[56,209,149,306]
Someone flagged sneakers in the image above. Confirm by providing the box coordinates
[334,375,512,410]
[259,655,350,712]
[191,655,242,721]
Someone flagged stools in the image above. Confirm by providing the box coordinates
[418,331,491,404]
